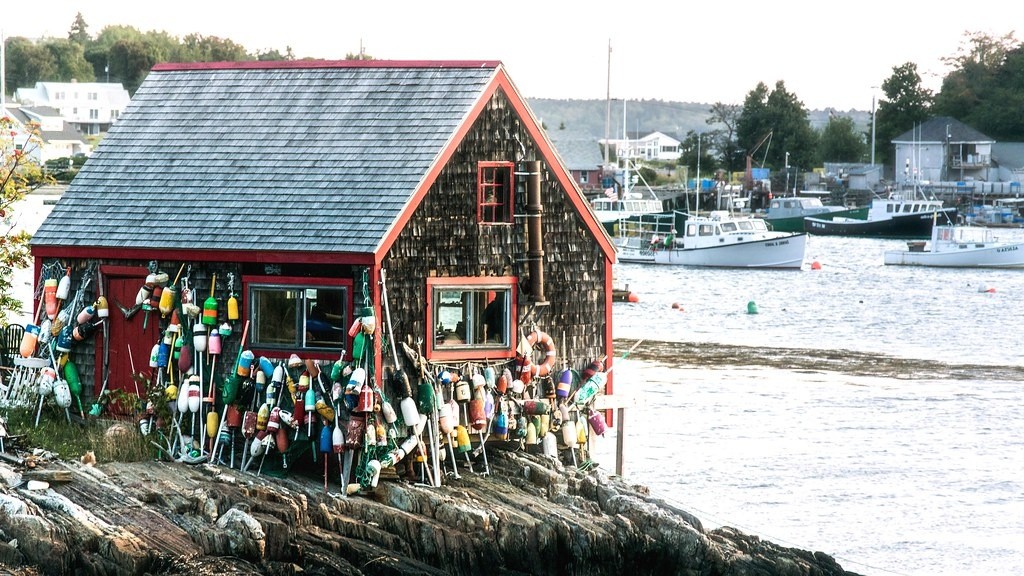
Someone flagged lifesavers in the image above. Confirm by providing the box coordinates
[522,331,557,376]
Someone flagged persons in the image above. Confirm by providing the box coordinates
[481,296,505,345]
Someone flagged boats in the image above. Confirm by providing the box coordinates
[589,35,1024,240]
[883,211,1024,269]
[615,131,808,271]
